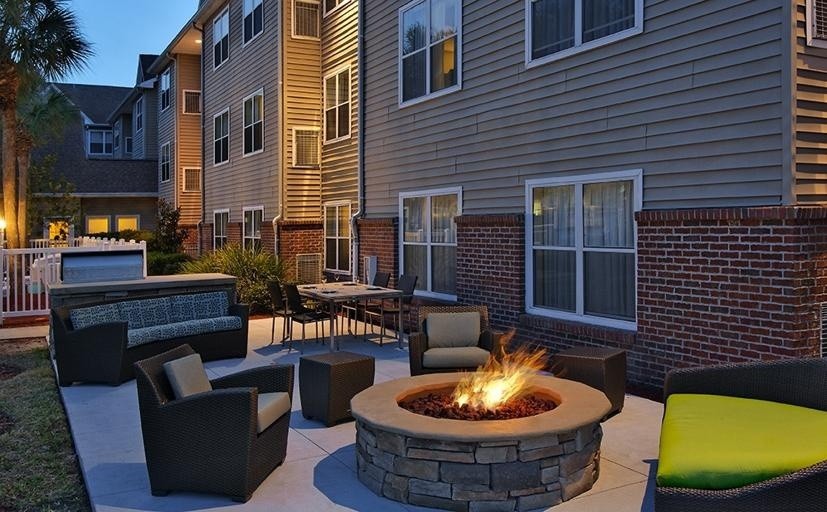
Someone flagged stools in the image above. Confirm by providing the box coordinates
[299,351,374,427]
[554,344,627,422]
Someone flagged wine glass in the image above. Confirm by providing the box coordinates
[354,276,360,291]
[334,271,341,285]
[321,275,327,288]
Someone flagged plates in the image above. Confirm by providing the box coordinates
[366,286,382,290]
[304,285,317,289]
[343,282,356,286]
[322,289,336,293]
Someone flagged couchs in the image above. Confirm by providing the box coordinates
[50,287,249,387]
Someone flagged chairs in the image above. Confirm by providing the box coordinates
[266,272,419,351]
[134,343,295,504]
[654,357,827,510]
[408,304,504,376]
